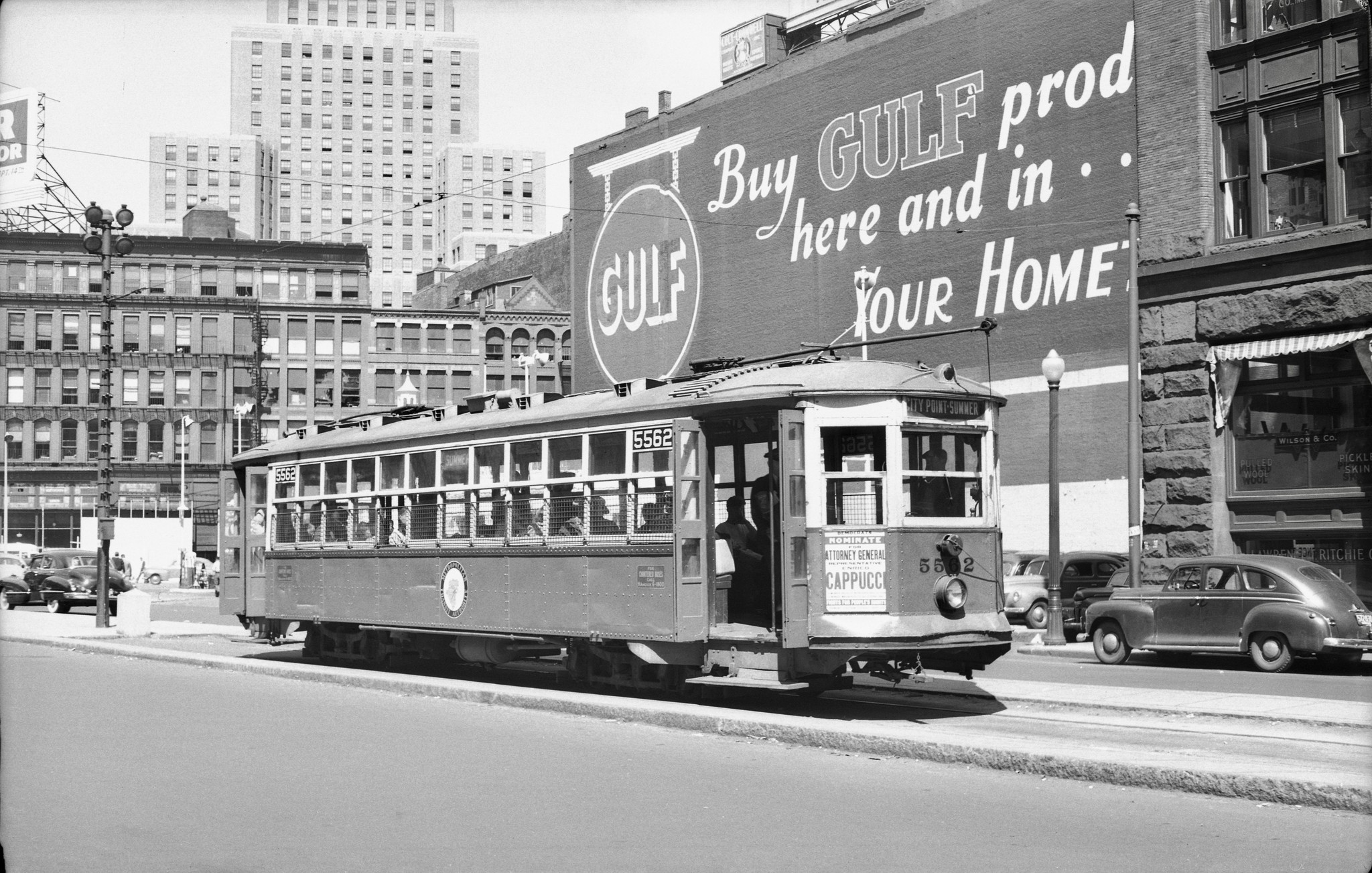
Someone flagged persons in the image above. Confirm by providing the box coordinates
[751,448,780,630]
[916,446,963,517]
[112,551,132,582]
[716,496,770,615]
[212,555,221,596]
[280,469,676,542]
[135,556,148,584]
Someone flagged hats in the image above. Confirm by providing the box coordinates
[764,448,781,461]
[573,496,609,515]
[921,448,948,458]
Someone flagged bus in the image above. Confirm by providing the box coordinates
[215,340,1017,687]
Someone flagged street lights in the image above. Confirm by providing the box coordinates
[234,401,255,455]
[1,432,15,543]
[517,348,550,396]
[855,264,877,362]
[1040,348,1074,649]
[82,203,134,627]
[180,414,194,520]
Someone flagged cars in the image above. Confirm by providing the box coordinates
[0,542,214,614]
[1001,548,1130,633]
[1076,553,1372,677]
